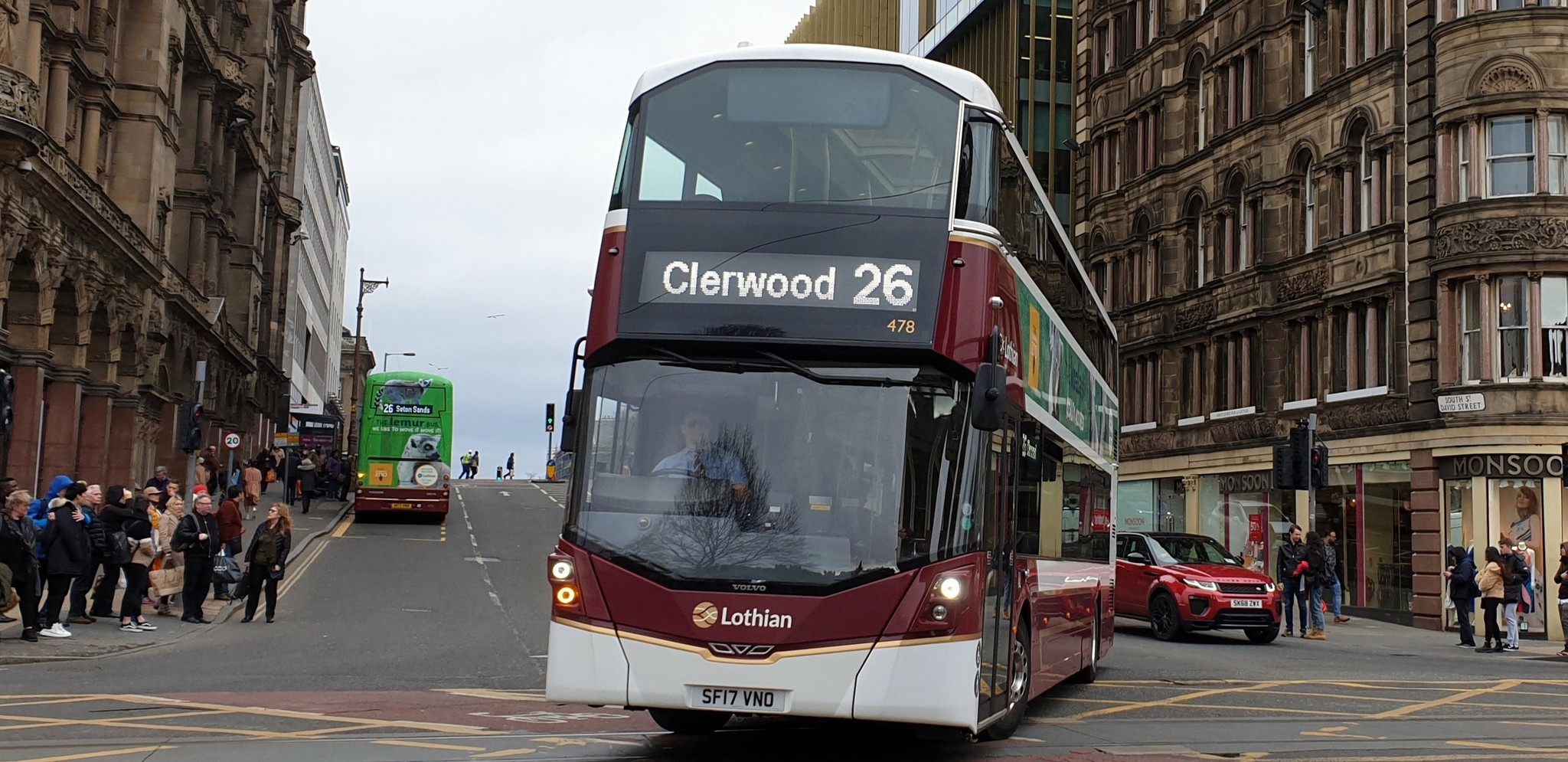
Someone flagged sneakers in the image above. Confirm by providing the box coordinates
[21,594,237,642]
[1282,616,1350,640]
[1454,639,1568,657]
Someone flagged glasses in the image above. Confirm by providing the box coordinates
[198,502,213,506]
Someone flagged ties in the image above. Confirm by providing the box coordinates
[695,453,706,478]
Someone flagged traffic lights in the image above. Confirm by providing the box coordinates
[179,402,205,450]
[497,470,502,480]
[546,403,554,432]
[1309,447,1329,489]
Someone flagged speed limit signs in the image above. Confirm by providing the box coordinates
[224,432,241,450]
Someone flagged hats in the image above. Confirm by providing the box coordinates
[144,487,163,495]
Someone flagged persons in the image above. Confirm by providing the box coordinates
[256,443,285,495]
[321,449,354,501]
[177,485,246,624]
[459,449,473,479]
[503,453,514,480]
[144,466,171,490]
[276,443,325,513]
[0,474,111,642]
[195,445,223,496]
[652,400,751,502]
[1443,538,1530,654]
[241,503,295,623]
[88,479,185,632]
[1500,486,1544,629]
[232,458,262,520]
[1554,542,1568,657]
[470,450,479,479]
[1276,525,1350,640]
[1175,545,1193,563]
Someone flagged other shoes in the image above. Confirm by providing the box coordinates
[339,498,349,502]
[245,514,250,520]
[302,509,309,513]
[252,509,257,519]
[267,617,274,623]
[241,617,252,622]
[290,497,294,505]
[0,613,17,623]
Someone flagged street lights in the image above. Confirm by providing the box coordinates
[383,353,416,373]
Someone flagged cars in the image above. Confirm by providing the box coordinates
[1111,530,1282,645]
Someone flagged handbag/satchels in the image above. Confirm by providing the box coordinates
[1444,579,1457,612]
[169,512,200,553]
[265,469,276,482]
[210,547,242,583]
[234,566,251,602]
[148,554,185,597]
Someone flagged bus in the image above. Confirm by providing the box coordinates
[547,43,1119,741]
[353,371,453,522]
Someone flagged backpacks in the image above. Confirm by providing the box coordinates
[111,520,146,564]
[1317,551,1337,589]
